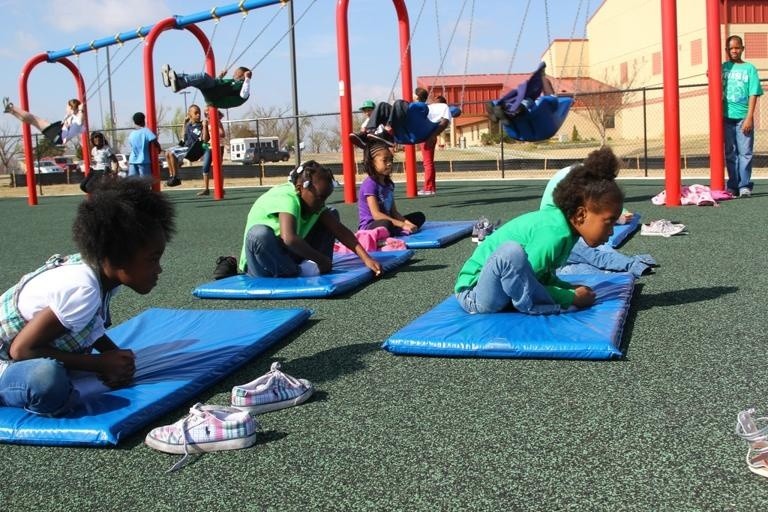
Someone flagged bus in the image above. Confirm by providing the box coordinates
[230,137,281,162]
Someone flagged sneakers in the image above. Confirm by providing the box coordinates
[640,219,685,237]
[740,188,752,198]
[728,189,738,196]
[472,217,494,242]
[367,128,394,147]
[417,191,431,196]
[231,370,313,416]
[161,64,180,93]
[214,256,237,278]
[165,177,180,187]
[349,132,367,149]
[3,98,13,113]
[197,190,209,196]
[145,404,257,454]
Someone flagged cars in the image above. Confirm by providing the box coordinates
[34,144,190,174]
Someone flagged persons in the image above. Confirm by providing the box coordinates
[238,160,384,278]
[540,145,633,225]
[485,69,555,126]
[358,140,425,238]
[161,64,252,108]
[454,165,624,314]
[80,105,227,196]
[3,97,84,146]
[349,88,453,196]
[706,35,764,198]
[0,176,177,417]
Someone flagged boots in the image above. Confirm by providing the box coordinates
[485,101,530,126]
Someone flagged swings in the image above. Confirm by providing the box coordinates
[202,1,316,107]
[187,12,249,159]
[50,40,142,186]
[384,0,475,142]
[502,0,588,141]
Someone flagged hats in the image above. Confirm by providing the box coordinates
[359,100,375,110]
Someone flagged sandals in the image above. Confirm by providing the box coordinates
[737,410,768,479]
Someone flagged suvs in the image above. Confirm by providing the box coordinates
[245,147,290,165]
